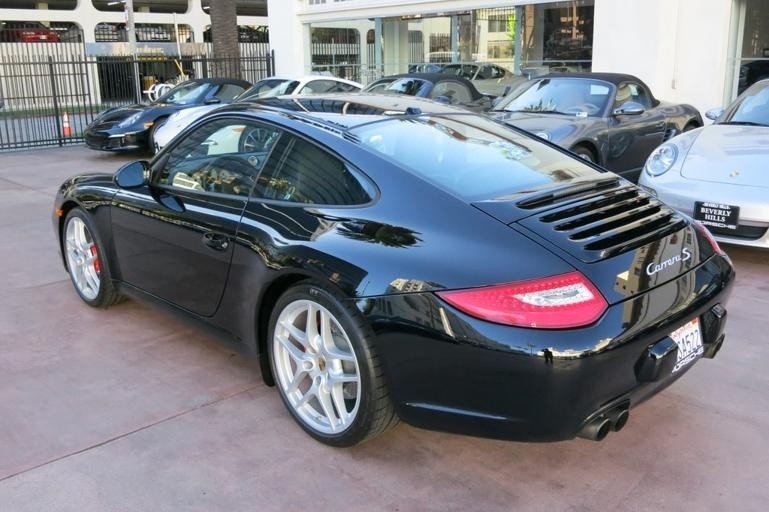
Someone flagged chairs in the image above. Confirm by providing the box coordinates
[612,86,633,113]
[283,143,348,210]
